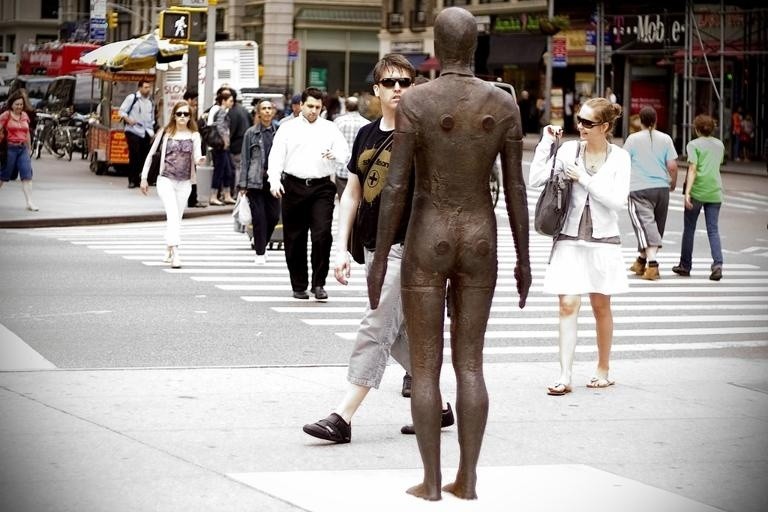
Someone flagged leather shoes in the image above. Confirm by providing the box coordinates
[171,255,182,268]
[187,198,238,208]
[26,205,39,211]
[293,291,311,300]
[309,285,328,300]
[165,248,174,263]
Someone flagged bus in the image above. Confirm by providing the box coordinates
[0,41,105,114]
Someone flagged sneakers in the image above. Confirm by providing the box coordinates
[671,263,691,276]
[302,412,352,445]
[642,264,660,280]
[399,403,454,435]
[710,267,723,281]
[629,257,646,276]
[255,249,267,267]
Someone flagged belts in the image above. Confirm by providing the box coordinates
[283,173,331,188]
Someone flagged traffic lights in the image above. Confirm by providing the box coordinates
[160,11,189,39]
[109,12,118,29]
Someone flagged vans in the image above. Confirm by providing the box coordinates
[199,92,288,119]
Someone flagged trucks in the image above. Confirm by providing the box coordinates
[110,39,262,119]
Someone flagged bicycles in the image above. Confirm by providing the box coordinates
[31,104,90,159]
[489,167,501,209]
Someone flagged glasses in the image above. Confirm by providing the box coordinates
[576,115,606,129]
[174,112,190,117]
[378,76,414,89]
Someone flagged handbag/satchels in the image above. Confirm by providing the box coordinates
[201,108,227,149]
[533,133,569,237]
[346,194,367,265]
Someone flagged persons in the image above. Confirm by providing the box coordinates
[517,83,617,138]
[671,114,725,282]
[620,103,679,280]
[0,96,39,212]
[366,8,533,504]
[300,53,456,443]
[731,107,755,163]
[528,96,634,394]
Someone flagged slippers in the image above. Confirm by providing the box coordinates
[547,382,572,396]
[586,377,615,388]
